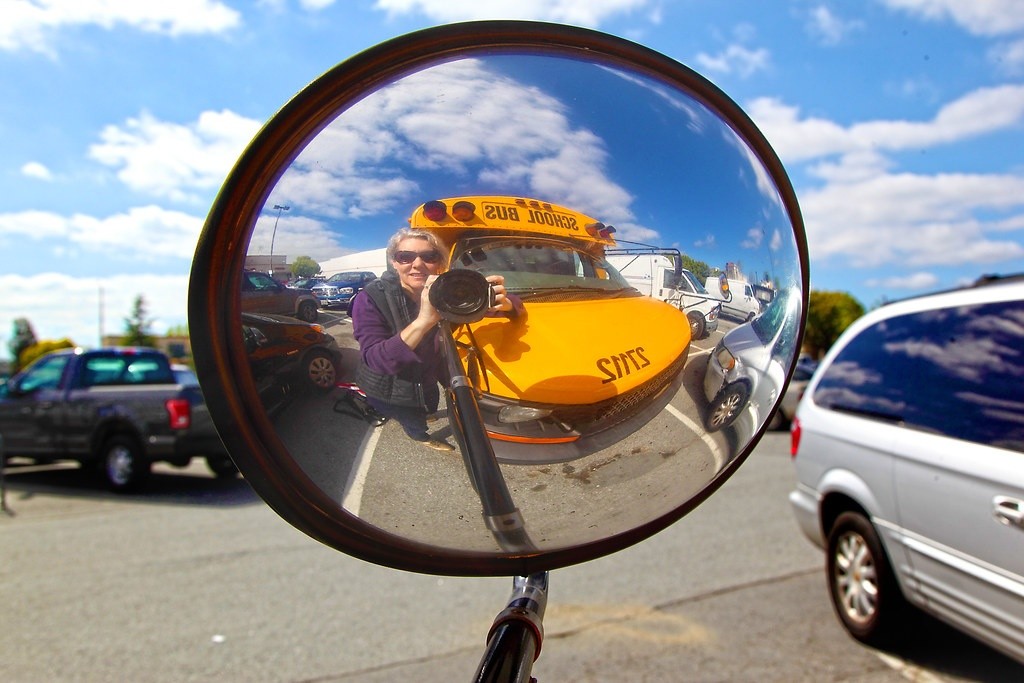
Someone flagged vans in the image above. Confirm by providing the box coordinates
[705,277,761,322]
[702,287,802,454]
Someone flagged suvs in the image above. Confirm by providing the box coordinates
[241,269,320,323]
[313,271,377,309]
[766,279,1024,664]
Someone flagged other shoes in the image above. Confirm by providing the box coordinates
[425,413,449,426]
[417,437,455,451]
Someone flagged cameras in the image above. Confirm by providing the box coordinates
[429,268,496,324]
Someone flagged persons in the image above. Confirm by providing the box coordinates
[349,229,513,451]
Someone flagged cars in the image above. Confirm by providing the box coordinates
[240,311,341,414]
[92,365,197,386]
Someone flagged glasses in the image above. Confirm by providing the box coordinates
[394,251,441,264]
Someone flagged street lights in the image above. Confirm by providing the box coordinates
[269,204,290,277]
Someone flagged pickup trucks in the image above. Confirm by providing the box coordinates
[1,347,249,491]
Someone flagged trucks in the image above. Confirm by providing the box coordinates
[607,253,722,338]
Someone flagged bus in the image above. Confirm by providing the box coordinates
[406,195,693,466]
